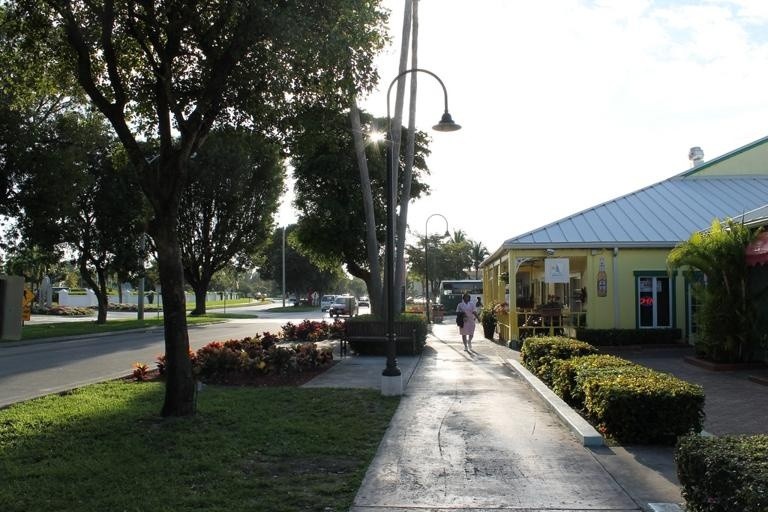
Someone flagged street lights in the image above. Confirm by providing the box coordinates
[282,213,301,308]
[382,69,462,376]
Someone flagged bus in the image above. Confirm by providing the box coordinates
[439,279,510,315]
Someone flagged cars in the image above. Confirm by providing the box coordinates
[320,294,370,318]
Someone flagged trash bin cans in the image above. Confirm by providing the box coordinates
[431,304,444,323]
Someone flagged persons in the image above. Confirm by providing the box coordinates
[473,297,483,320]
[455,293,481,351]
